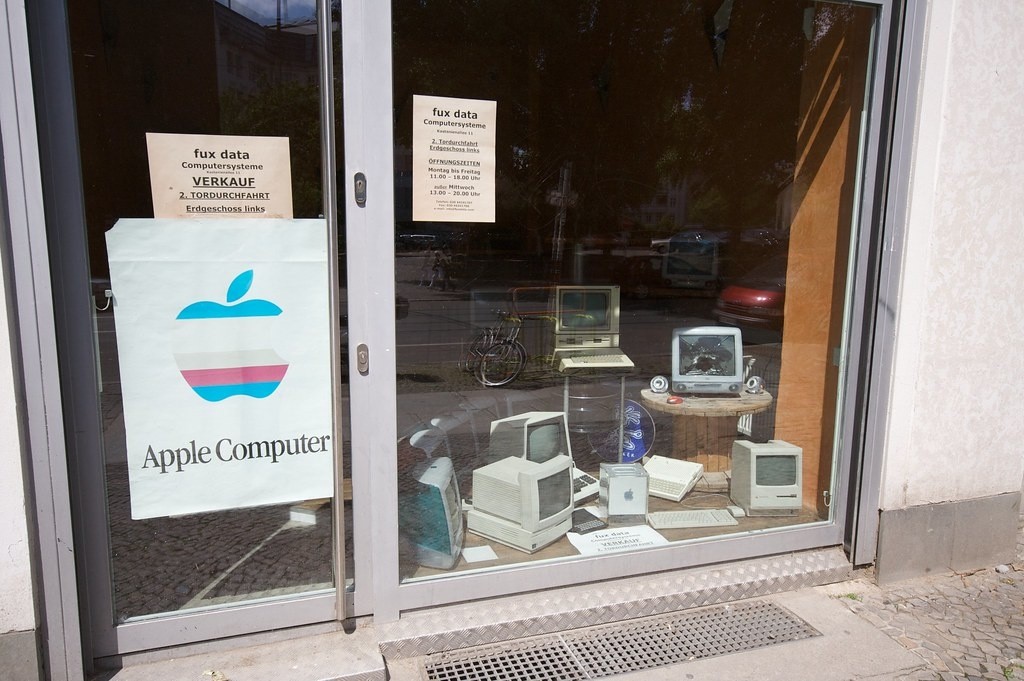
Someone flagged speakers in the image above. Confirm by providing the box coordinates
[747,375,766,394]
[651,376,669,393]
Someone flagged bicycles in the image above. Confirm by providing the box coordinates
[466,307,552,386]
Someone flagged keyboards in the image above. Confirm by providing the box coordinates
[573,474,600,508]
[553,350,635,373]
[648,509,739,530]
[649,477,686,499]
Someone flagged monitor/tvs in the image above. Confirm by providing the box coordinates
[398,456,464,569]
[542,285,620,349]
[488,411,573,464]
[672,326,743,393]
[730,440,804,516]
[467,454,574,554]
[664,238,719,290]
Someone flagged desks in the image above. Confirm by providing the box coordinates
[559,367,638,463]
[639,381,774,494]
[621,283,719,319]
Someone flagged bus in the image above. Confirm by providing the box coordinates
[651,234,719,299]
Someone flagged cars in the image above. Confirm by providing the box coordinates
[715,270,785,344]
